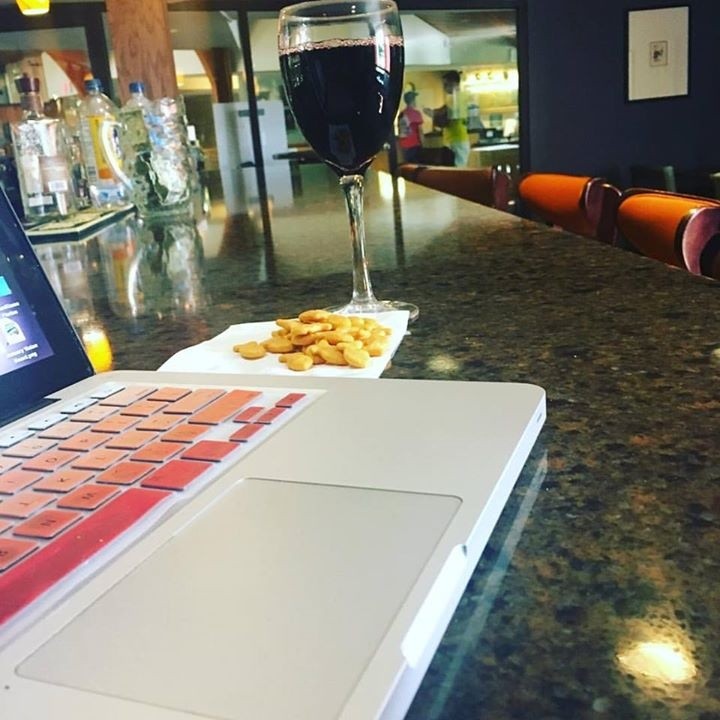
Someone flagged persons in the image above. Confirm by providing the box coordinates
[423,70,470,166]
[398,92,424,164]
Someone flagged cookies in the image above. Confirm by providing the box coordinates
[233,310,392,370]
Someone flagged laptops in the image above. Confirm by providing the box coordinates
[0,180,548,720]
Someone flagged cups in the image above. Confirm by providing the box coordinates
[113,96,189,219]
[138,218,215,315]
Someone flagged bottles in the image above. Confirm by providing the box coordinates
[34,245,92,348]
[74,78,133,209]
[48,95,91,212]
[97,224,150,337]
[120,83,150,111]
[12,74,72,217]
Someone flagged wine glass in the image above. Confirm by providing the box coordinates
[278,0,420,322]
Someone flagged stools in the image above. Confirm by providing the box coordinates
[393,162,720,282]
